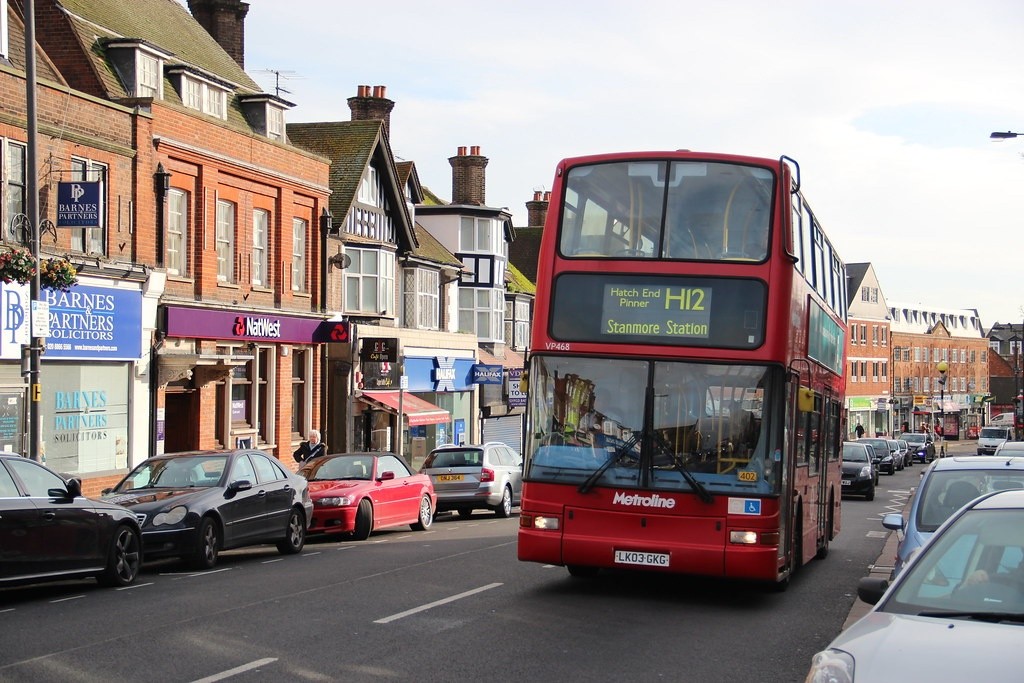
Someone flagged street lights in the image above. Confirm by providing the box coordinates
[992,325,1019,441]
[937,360,948,457]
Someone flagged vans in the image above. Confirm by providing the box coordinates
[991,413,1014,427]
[977,426,1012,456]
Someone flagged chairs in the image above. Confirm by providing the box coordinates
[942,481,980,508]
[196,472,221,484]
[338,463,350,475]
[349,464,366,476]
[171,471,192,483]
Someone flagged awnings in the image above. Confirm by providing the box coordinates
[912,411,930,414]
[362,390,451,426]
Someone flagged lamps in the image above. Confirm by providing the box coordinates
[320,207,332,239]
[155,162,172,203]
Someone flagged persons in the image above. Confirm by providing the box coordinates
[854,422,864,438]
[581,389,626,433]
[292,429,328,470]
[901,420,909,433]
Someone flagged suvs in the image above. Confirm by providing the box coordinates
[417,441,523,519]
[898,433,936,463]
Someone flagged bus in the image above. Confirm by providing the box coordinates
[516,148,852,599]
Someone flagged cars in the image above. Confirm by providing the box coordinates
[995,442,1024,457]
[881,456,1024,599]
[804,487,1024,683]
[897,439,914,467]
[863,444,880,487]
[0,453,143,591]
[295,452,437,542]
[857,437,895,475]
[888,441,905,471]
[100,449,313,570]
[841,442,880,501]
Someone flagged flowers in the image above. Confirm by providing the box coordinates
[0,247,37,286]
[40,257,79,294]
